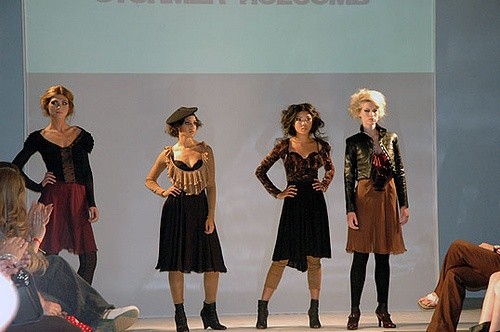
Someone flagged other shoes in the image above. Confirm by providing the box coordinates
[96,306,140,332]
[469,322,491,332]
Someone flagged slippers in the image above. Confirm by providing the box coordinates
[417,292,439,310]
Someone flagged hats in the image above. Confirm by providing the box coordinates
[166,107,197,125]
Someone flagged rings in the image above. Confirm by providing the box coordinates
[55,308,59,311]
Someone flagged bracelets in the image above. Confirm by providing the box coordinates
[31,237,41,243]
[492,244,499,253]
[0,253,18,266]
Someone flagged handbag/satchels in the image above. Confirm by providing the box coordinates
[0,254,43,326]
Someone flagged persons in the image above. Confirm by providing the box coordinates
[255,104,336,329]
[414,240,500,332]
[344,89,409,331]
[145,106,227,332]
[0,85,140,332]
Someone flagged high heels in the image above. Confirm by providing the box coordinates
[175,304,189,332]
[200,301,226,330]
[308,299,321,328]
[347,312,360,330]
[376,307,396,328]
[256,300,268,329]
